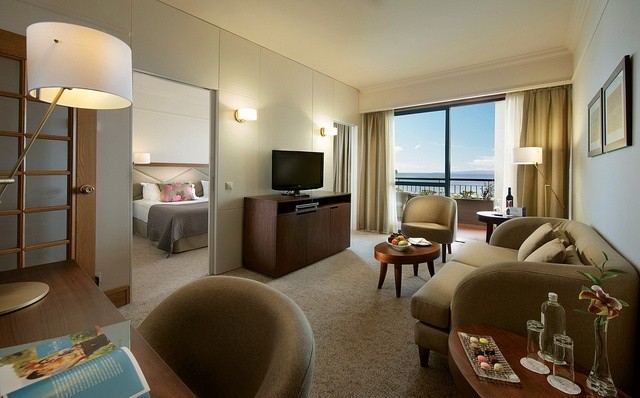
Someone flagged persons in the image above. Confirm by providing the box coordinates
[26,333,112,380]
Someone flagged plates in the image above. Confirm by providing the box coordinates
[537,350,567,365]
[520,357,550,375]
[547,375,582,395]
[457,331,521,384]
[409,237,432,246]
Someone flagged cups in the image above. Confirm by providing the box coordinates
[525,319,545,369]
[552,334,575,387]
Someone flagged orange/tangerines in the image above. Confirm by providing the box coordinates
[392,239,398,245]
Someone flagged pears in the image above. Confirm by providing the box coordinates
[398,240,408,246]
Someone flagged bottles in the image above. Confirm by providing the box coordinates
[539,292,566,360]
[506,187,513,215]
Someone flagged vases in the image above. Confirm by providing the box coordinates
[586,315,617,398]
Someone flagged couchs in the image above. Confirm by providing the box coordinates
[401,196,458,263]
[409,216,640,391]
[135,275,317,398]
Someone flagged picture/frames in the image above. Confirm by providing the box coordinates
[601,55,631,154]
[588,88,603,158]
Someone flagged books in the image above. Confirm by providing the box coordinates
[0,320,151,398]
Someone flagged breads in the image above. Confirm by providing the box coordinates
[473,349,485,356]
[470,341,480,348]
[470,336,478,343]
[489,354,497,362]
[481,361,491,372]
[485,347,497,356]
[478,354,487,362]
[493,363,504,372]
[479,337,488,344]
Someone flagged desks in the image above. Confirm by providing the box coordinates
[477,211,513,244]
[1,259,196,398]
[447,326,631,398]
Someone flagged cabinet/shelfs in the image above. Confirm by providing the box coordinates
[243,190,351,278]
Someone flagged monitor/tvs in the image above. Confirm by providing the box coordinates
[272,150,324,197]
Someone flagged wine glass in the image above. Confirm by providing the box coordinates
[494,198,502,213]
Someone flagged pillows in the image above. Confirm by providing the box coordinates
[553,226,570,247]
[521,238,567,265]
[157,183,194,202]
[517,222,553,261]
[141,183,160,199]
[565,245,585,266]
[201,180,209,197]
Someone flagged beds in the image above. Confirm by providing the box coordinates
[132,163,209,253]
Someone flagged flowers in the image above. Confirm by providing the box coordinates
[575,252,631,375]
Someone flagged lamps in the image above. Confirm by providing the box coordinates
[235,108,258,123]
[134,152,151,164]
[513,146,567,210]
[0,20,135,196]
[321,127,338,137]
